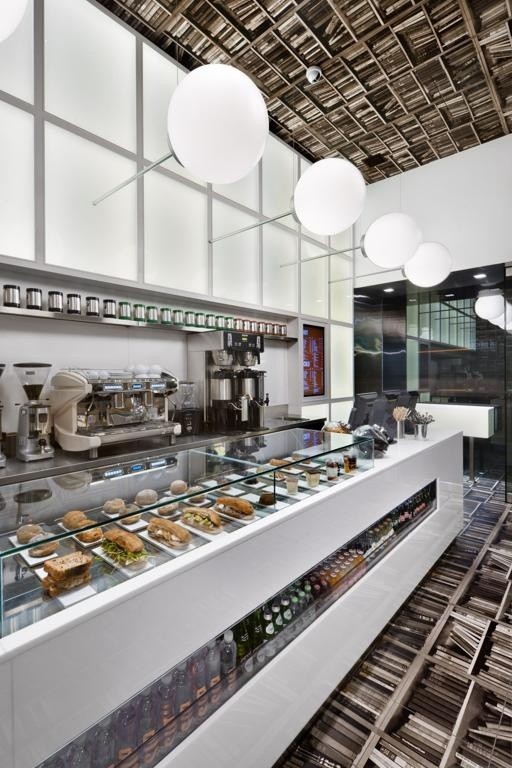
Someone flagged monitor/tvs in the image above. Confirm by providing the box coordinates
[348,392,386,426]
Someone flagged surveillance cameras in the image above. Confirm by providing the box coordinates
[306,66,322,84]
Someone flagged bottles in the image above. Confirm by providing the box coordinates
[2,284,288,340]
[34,482,436,767]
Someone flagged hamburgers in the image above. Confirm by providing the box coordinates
[43,495,253,597]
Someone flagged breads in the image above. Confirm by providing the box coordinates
[270,459,292,469]
[260,494,274,505]
[244,477,258,484]
[292,454,310,464]
[256,467,265,473]
[15,479,205,557]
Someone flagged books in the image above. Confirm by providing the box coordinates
[93,0,512,187]
[270,473,511,766]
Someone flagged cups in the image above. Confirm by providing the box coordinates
[285,451,359,495]
[395,420,406,438]
[412,423,428,439]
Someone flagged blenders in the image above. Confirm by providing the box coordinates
[12,361,58,464]
[173,381,203,440]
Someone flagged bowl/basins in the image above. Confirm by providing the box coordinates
[52,362,178,388]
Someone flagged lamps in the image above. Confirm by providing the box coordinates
[1,0,27,43]
[210,157,366,244]
[419,295,512,331]
[281,212,422,269]
[329,241,452,289]
[91,63,269,205]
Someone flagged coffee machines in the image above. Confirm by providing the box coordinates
[185,331,272,433]
[47,360,183,459]
[0,363,7,467]
